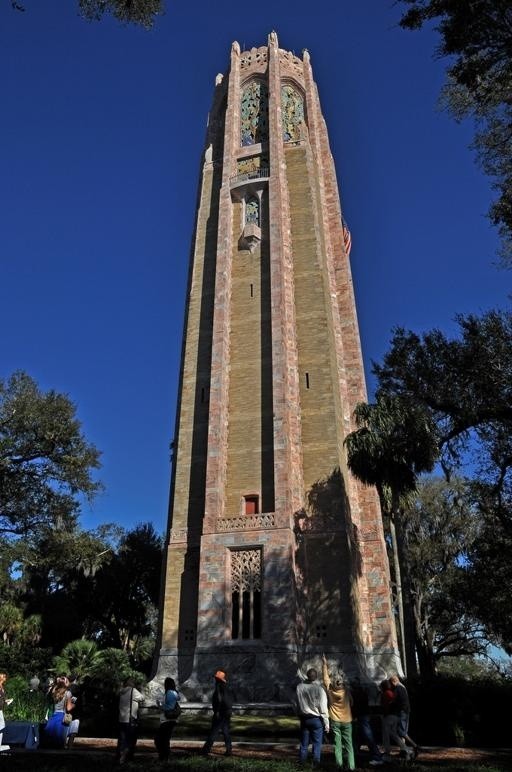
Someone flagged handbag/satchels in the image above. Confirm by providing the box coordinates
[164,702,181,720]
[129,718,140,728]
[63,713,72,724]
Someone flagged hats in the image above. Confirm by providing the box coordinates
[215,671,226,684]
[331,674,343,689]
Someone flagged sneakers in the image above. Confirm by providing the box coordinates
[382,754,391,761]
[369,760,383,765]
[400,747,421,761]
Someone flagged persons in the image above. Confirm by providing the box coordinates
[347,676,384,766]
[390,675,422,757]
[115,676,146,762]
[0,670,81,753]
[295,668,330,762]
[154,677,181,765]
[198,670,233,754]
[320,650,356,772]
[378,679,416,762]
